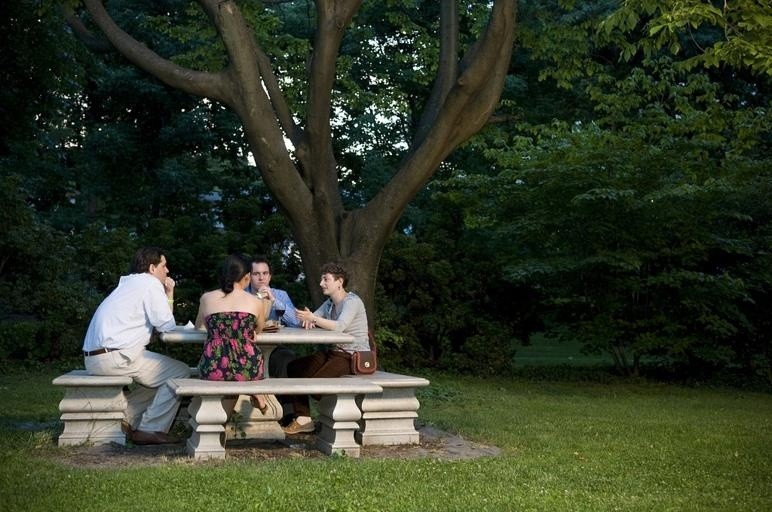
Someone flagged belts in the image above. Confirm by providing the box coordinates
[82,347,119,357]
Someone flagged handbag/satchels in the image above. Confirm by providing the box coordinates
[349,349,378,375]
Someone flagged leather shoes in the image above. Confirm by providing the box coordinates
[131,429,180,446]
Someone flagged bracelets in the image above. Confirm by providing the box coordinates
[312,315,317,323]
[167,298,174,303]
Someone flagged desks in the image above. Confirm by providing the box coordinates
[158,325,355,446]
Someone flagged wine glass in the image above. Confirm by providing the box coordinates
[275,303,285,322]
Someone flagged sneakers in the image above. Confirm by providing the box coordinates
[281,419,315,434]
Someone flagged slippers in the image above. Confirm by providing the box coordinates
[249,396,269,416]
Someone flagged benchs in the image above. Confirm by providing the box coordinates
[50,367,134,450]
[165,377,383,462]
[343,369,431,446]
[188,365,199,376]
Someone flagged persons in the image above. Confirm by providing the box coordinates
[244,255,304,405]
[193,255,268,447]
[281,260,370,435]
[81,247,185,444]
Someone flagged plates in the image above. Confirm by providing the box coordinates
[263,325,279,334]
